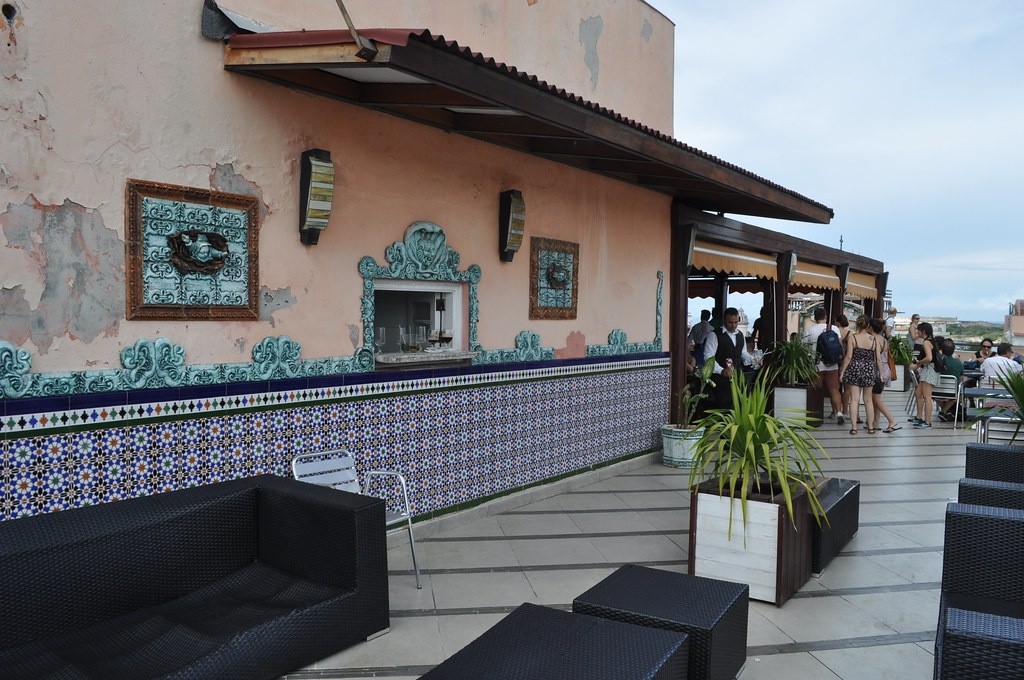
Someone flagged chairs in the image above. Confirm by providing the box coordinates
[292,449,421,589]
[904,367,1024,680]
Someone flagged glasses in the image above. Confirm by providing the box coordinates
[914,318,920,320]
[983,345,991,348]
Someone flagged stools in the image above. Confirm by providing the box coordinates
[573,564,749,680]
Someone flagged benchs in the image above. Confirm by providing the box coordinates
[967,408,1024,444]
[795,474,860,578]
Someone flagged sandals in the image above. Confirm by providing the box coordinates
[868,429,875,434]
[850,429,857,434]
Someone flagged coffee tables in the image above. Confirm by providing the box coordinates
[415,602,688,680]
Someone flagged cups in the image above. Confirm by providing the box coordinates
[402,334,422,354]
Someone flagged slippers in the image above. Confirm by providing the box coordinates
[863,425,882,430]
[883,427,903,432]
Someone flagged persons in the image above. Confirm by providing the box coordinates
[686,307,1022,434]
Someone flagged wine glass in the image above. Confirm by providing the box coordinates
[374,326,388,356]
[396,325,454,353]
[749,350,763,369]
[725,358,735,381]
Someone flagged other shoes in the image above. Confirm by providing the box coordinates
[938,412,949,421]
[857,417,863,423]
[946,412,955,421]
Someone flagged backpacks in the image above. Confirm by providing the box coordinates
[934,353,946,372]
[962,360,980,370]
[813,323,844,367]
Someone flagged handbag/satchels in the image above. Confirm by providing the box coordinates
[906,334,916,350]
[888,353,897,381]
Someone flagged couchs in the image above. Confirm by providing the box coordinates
[0,474,391,680]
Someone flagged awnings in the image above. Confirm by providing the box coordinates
[223,29,835,225]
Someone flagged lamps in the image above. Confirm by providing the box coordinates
[499,189,525,262]
[300,148,335,245]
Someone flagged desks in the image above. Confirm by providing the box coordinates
[963,370,984,388]
[964,388,1014,406]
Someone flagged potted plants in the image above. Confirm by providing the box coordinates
[882,334,912,391]
[662,329,832,608]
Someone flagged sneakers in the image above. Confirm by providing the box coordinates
[907,417,923,423]
[836,412,844,424]
[829,414,837,420]
[913,422,932,429]
